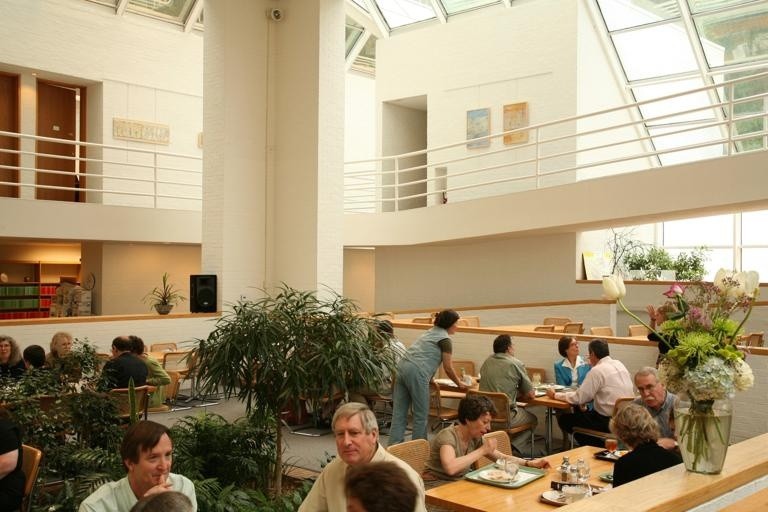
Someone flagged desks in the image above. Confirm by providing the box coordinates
[424,446,630,511]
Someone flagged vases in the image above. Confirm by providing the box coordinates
[670,394,732,473]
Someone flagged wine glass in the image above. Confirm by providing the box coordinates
[605,438,617,457]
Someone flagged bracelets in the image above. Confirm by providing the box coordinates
[649,316,656,323]
[674,441,679,450]
[525,460,528,466]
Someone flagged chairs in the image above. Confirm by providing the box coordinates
[735,332,763,347]
[90,341,239,414]
[477,430,512,469]
[385,438,431,474]
[109,386,150,422]
[353,312,647,340]
[19,444,42,511]
[289,360,640,458]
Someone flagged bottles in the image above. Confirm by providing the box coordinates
[460,368,465,380]
[560,456,590,483]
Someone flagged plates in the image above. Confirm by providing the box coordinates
[599,472,613,482]
[614,449,629,456]
[543,490,567,506]
[478,470,518,483]
[541,384,565,389]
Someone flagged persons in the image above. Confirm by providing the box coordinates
[632,366,683,455]
[644,305,679,366]
[296,402,426,512]
[0,408,27,512]
[479,334,537,458]
[388,309,470,447]
[421,395,551,490]
[79,422,198,512]
[127,492,193,512]
[554,336,595,432]
[0,332,172,433]
[546,340,635,445]
[609,402,683,489]
[345,461,419,512]
[373,320,408,429]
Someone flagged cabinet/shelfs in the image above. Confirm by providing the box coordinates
[0,280,40,320]
[38,283,60,318]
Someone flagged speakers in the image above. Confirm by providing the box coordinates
[190,275,217,312]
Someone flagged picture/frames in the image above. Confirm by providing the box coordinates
[466,107,491,148]
[503,100,528,145]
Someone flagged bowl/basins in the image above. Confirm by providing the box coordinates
[563,488,587,499]
[496,459,515,470]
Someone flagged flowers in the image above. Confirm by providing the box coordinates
[596,263,759,462]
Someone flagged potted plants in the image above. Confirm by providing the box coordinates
[142,271,187,315]
